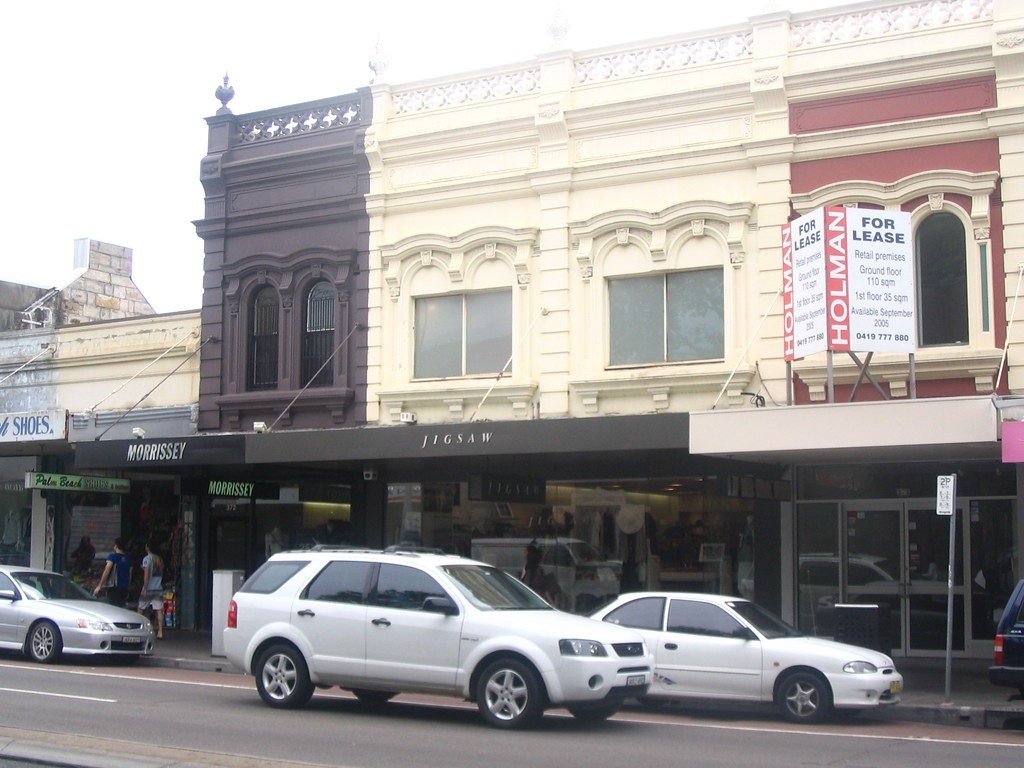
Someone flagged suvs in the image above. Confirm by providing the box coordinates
[989,578,1024,700]
[784,551,912,625]
[222,544,654,733]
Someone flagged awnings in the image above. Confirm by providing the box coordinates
[72,395,1003,480]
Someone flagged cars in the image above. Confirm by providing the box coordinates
[0,564,157,666]
[585,592,904,719]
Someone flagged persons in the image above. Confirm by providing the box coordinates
[0,508,24,564]
[94,537,131,607]
[264,525,290,561]
[136,540,165,639]
[72,535,96,574]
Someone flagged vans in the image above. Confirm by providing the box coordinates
[470,537,623,613]
